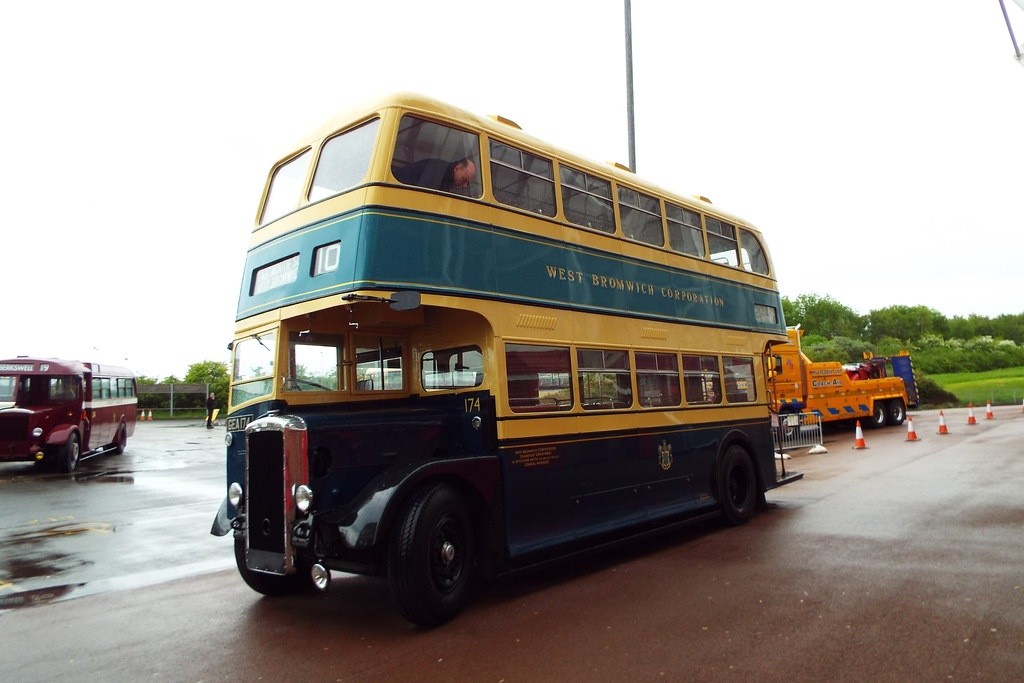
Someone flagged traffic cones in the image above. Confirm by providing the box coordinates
[984,399,996,421]
[147,408,153,421]
[139,408,146,421]
[966,402,979,425]
[936,410,952,434]
[905,414,921,441]
[852,419,870,449]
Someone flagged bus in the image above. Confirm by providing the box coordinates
[210,90,790,629]
[0,354,139,475]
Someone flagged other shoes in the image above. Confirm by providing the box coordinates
[207,425,214,429]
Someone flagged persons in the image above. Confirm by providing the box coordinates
[712,362,739,395]
[393,157,477,192]
[206,392,215,429]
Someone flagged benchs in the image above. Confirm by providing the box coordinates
[627,394,676,405]
[557,397,615,411]
[595,397,653,407]
[509,398,560,413]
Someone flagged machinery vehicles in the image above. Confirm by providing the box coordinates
[707,323,920,443]
